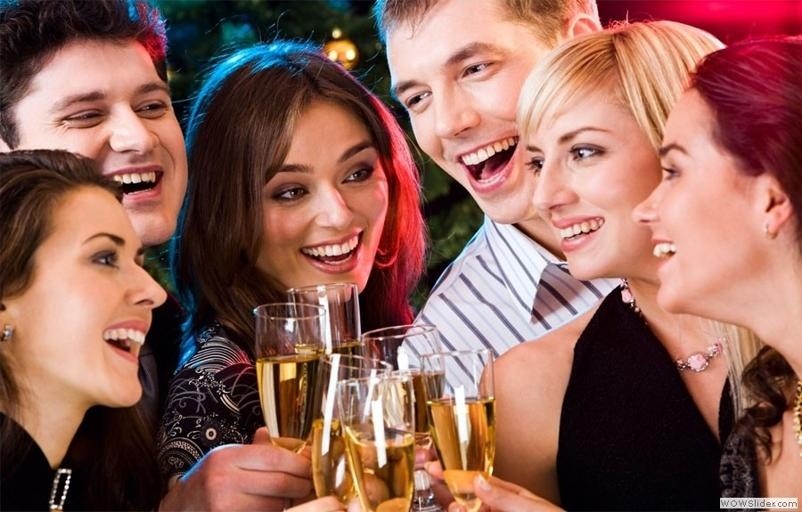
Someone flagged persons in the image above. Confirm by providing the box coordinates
[448,36,801,512]
[0,148,345,512]
[157,40,427,512]
[413,19,769,512]
[371,0,607,512]
[0,0,315,512]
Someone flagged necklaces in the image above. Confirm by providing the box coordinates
[789,379,802,445]
[617,278,723,372]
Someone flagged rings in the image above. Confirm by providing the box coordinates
[279,495,293,510]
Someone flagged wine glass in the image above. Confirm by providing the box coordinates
[251,281,501,512]
